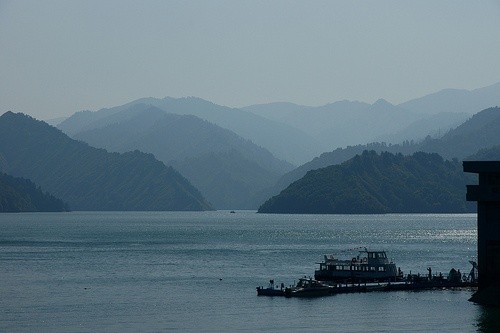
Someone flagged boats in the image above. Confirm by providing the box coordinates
[284,278,333,298]
[315,246,402,282]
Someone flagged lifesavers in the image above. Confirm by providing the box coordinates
[352,258,356,262]
[363,257,367,262]
